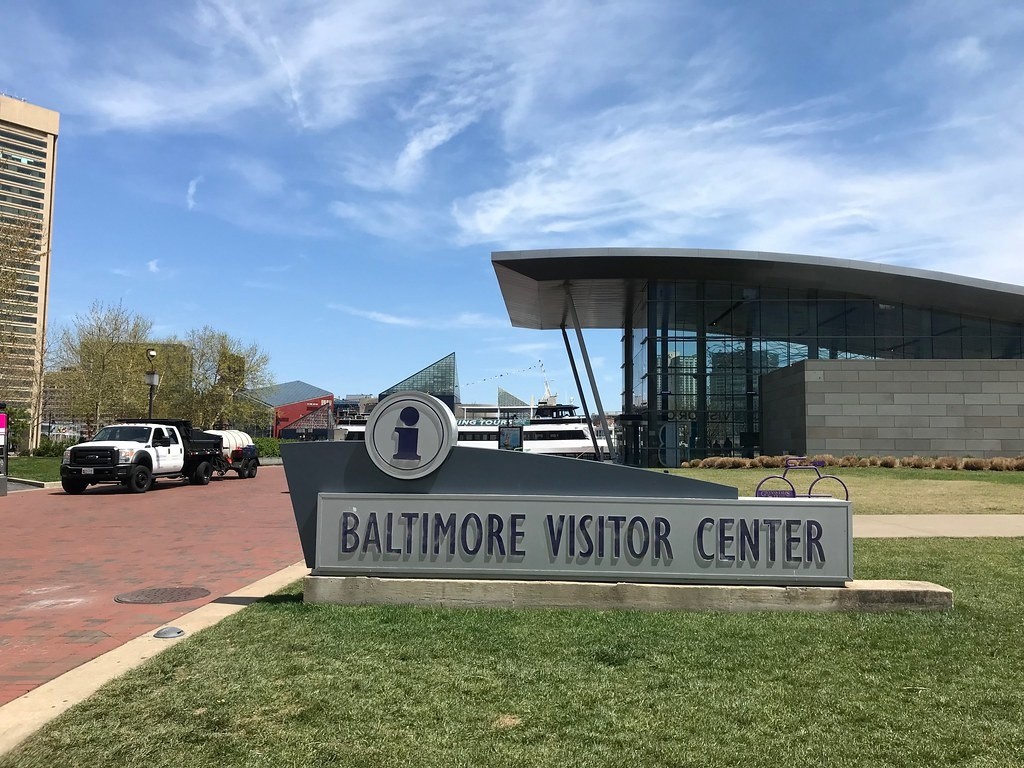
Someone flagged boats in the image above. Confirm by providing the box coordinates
[333,360,601,460]
[591,411,697,464]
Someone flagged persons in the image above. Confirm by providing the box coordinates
[713,437,732,456]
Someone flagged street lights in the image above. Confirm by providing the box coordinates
[145,348,160,418]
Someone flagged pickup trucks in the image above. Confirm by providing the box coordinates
[59,418,223,491]
